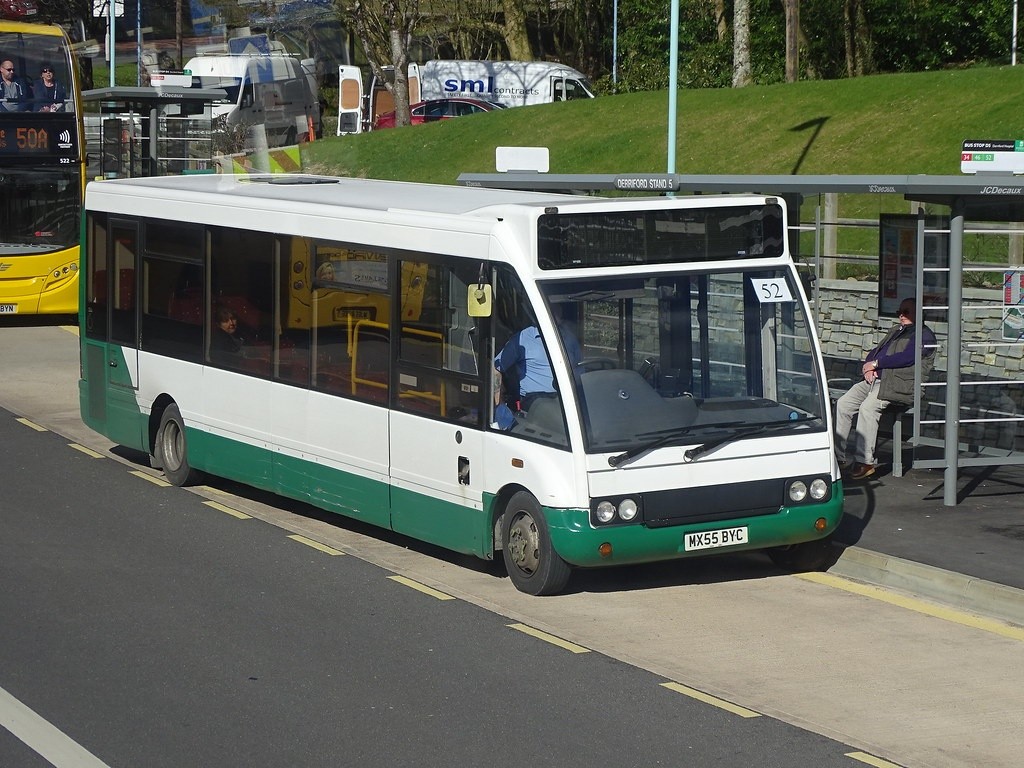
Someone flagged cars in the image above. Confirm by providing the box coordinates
[372,98,503,131]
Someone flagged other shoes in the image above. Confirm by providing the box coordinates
[849,462,875,479]
[836,459,844,464]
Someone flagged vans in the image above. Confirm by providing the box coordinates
[336,59,597,137]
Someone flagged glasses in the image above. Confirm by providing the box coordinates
[897,310,914,316]
[2,67,15,72]
[41,68,52,73]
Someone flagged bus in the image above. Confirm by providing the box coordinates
[86,209,429,336]
[0,16,90,315]
[79,173,846,597]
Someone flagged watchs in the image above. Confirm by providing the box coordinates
[872,360,878,369]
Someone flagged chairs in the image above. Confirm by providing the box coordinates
[32,63,52,83]
[92,270,476,416]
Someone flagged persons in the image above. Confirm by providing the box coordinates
[833,297,938,480]
[209,306,262,371]
[493,300,586,413]
[0,59,29,113]
[31,65,66,113]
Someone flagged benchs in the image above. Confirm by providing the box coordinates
[692,364,915,477]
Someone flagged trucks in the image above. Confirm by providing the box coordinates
[158,56,320,158]
